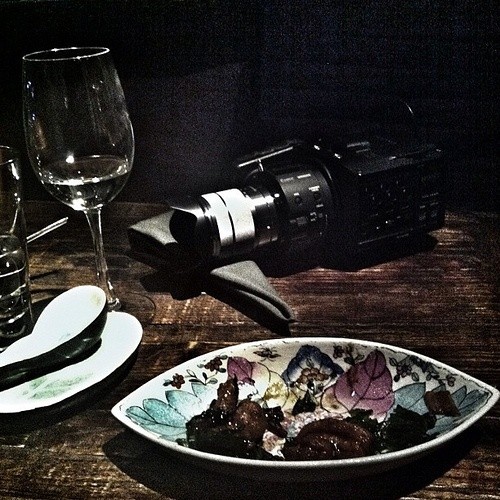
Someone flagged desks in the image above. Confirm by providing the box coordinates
[0,192,500,500]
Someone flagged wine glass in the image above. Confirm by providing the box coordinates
[19,44,156,329]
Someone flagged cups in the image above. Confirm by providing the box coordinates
[0,146,29,329]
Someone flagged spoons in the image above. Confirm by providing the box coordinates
[0,285,110,374]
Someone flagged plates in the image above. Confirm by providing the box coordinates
[111,335,500,479]
[0,310,147,418]
